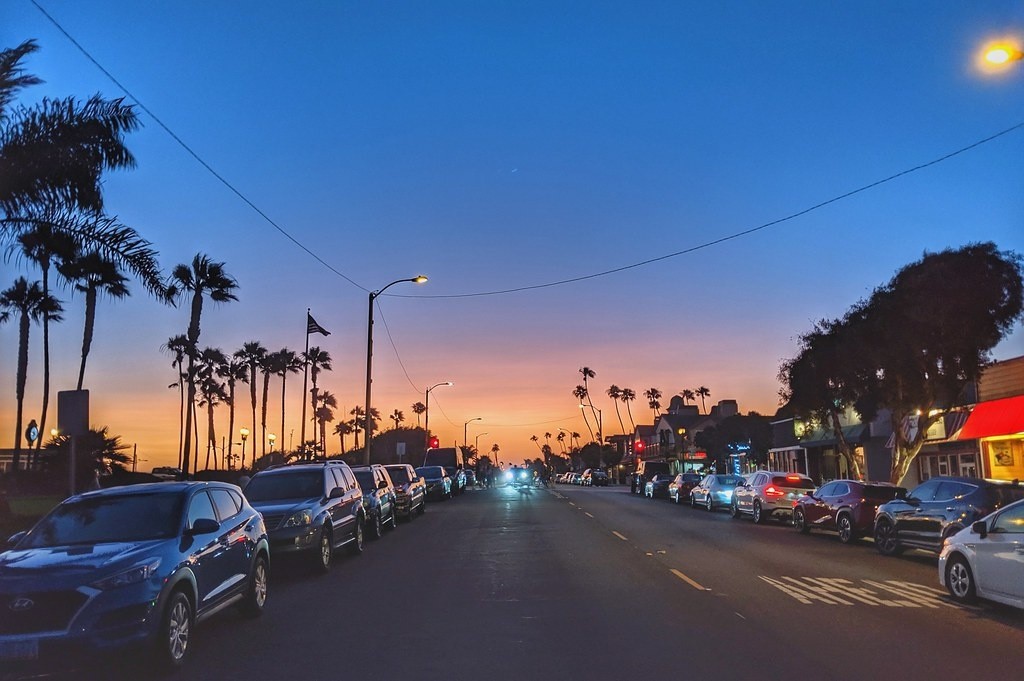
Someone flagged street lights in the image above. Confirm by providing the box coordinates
[240,427,250,469]
[268,434,277,466]
[579,404,602,469]
[475,433,488,481]
[221,435,242,474]
[558,428,573,467]
[463,417,482,469]
[678,428,686,473]
[364,275,429,466]
[425,382,454,455]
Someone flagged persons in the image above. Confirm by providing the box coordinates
[471,465,495,491]
[510,462,556,489]
[237,467,250,490]
[86,469,100,492]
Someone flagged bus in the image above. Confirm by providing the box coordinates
[423,447,467,497]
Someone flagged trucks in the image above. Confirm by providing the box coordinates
[631,460,671,496]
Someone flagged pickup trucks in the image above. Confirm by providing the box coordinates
[381,463,426,522]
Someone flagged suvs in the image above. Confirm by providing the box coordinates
[349,462,397,540]
[0,479,270,681]
[239,459,372,574]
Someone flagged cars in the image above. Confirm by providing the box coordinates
[729,470,821,525]
[792,479,908,545]
[872,475,1024,563]
[416,465,453,501]
[689,473,748,512]
[667,473,707,504]
[465,469,477,486]
[505,467,528,485]
[553,468,610,487]
[937,497,1024,618]
[643,471,676,500]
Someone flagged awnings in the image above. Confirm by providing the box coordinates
[798,423,870,447]
[885,403,974,450]
[955,395,1024,443]
